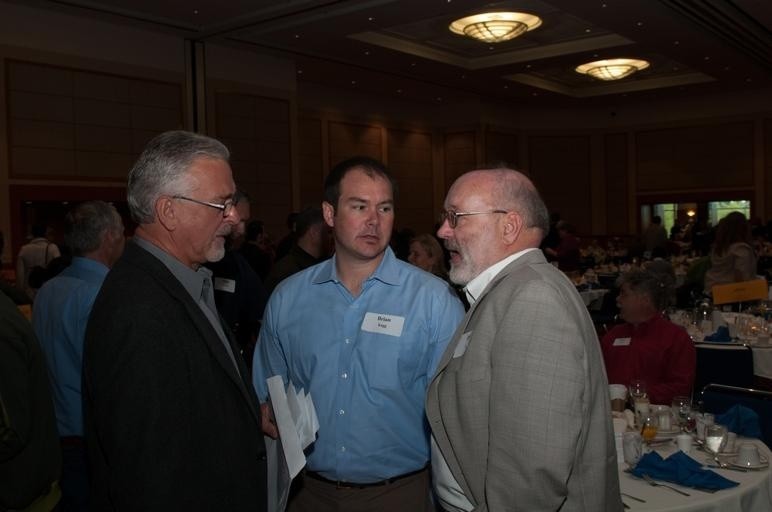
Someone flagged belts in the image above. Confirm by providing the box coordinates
[305,470,426,488]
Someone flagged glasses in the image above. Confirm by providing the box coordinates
[442,210,508,228]
[173,194,238,218]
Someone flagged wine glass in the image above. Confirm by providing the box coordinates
[622,377,727,474]
[676,310,771,345]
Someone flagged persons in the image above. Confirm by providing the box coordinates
[16,224,60,289]
[235,221,273,291]
[682,214,715,258]
[212,203,270,376]
[247,152,467,512]
[575,235,633,271]
[601,268,695,412]
[408,233,455,292]
[750,215,764,240]
[320,221,335,261]
[538,212,582,270]
[31,199,126,512]
[425,165,628,511]
[272,208,321,290]
[564,270,589,292]
[0,290,60,512]
[80,126,283,512]
[644,216,666,249]
[704,211,761,318]
[670,218,681,237]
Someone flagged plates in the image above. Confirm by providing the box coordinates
[723,455,770,472]
[655,424,680,433]
[594,265,616,272]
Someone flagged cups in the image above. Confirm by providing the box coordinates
[676,435,691,454]
[735,442,761,466]
